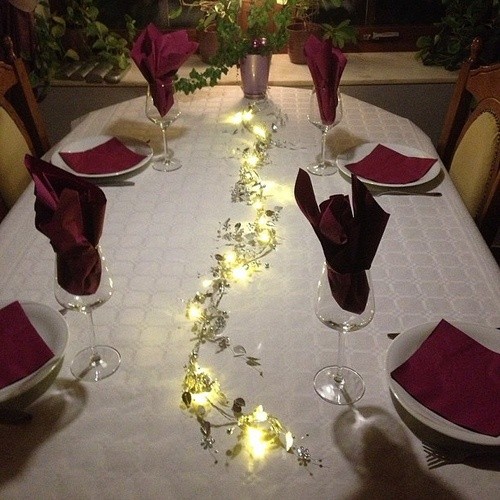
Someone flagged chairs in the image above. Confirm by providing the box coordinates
[436,38,500,248]
[0,36,51,225]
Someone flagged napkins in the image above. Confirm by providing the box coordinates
[391,319,500,438]
[302,33,348,124]
[130,23,201,117]
[23,153,107,297]
[345,144,439,185]
[58,136,146,174]
[294,167,390,314]
[0,299,54,390]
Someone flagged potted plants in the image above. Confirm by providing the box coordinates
[27,0,131,101]
[244,0,357,76]
[414,0,500,72]
[168,0,260,96]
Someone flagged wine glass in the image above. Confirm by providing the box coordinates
[54,244,122,382]
[145,80,184,172]
[313,260,375,407]
[307,86,344,177]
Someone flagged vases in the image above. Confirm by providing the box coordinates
[240,38,272,99]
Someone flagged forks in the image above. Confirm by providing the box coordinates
[421,438,500,459]
[370,189,443,198]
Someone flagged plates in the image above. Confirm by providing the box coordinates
[51,134,153,178]
[336,142,441,188]
[385,319,500,447]
[0,298,71,403]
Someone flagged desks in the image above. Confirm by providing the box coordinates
[0,85,500,500]
